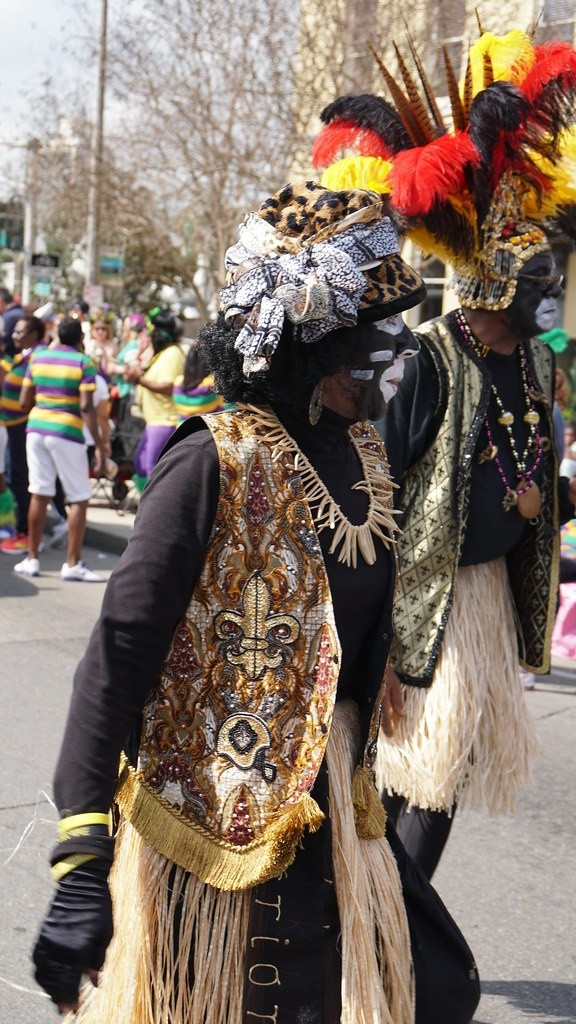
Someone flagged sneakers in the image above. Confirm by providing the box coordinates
[61,559,106,582]
[13,556,40,576]
[0,533,44,555]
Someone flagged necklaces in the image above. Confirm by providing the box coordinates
[237,401,405,569]
[454,310,543,512]
[458,309,540,524]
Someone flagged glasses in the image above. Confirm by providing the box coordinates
[518,274,566,296]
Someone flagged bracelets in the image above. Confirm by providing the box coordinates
[50,813,116,882]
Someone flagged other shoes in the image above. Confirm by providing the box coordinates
[520,673,535,690]
[50,516,68,547]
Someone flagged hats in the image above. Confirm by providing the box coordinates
[218,181,427,383]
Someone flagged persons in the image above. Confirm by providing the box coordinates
[0,286,576,690]
[378,217,576,886]
[29,178,482,1024]
